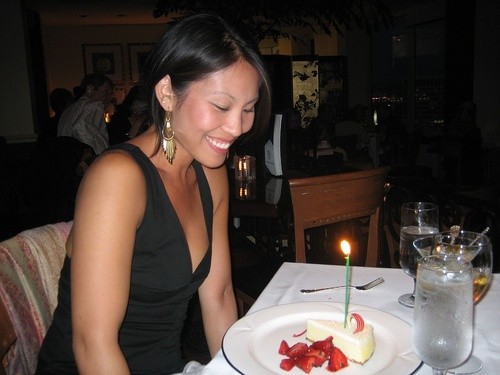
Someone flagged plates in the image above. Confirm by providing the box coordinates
[221,301,425,375]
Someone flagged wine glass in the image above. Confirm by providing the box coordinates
[430,231,493,375]
[398,203,439,307]
[412,254,475,375]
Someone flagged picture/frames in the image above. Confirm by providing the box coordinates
[127,42,157,80]
[81,43,124,86]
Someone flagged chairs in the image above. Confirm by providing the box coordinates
[0,220,74,375]
[289,166,391,268]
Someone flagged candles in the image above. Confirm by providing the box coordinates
[340,240,351,327]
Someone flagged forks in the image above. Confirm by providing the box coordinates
[300,278,384,292]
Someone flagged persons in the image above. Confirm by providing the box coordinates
[287,105,408,174]
[38,72,153,157]
[33,14,271,375]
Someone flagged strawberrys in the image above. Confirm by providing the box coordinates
[278,336,347,373]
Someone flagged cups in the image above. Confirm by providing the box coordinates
[413,236,442,257]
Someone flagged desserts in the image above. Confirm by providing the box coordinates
[306,313,376,365]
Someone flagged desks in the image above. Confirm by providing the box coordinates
[230,160,354,239]
[202,262,500,375]
